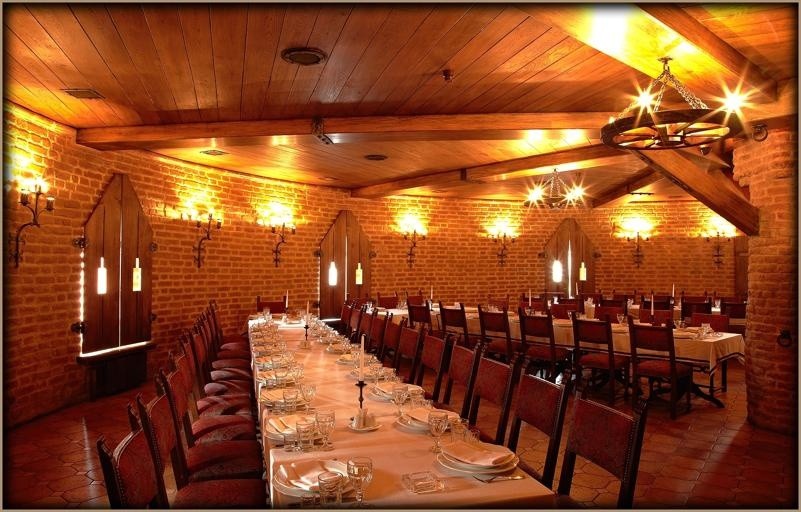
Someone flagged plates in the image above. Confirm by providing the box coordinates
[403,471,446,495]
[369,384,422,400]
[349,422,382,433]
[394,414,429,431]
[265,419,323,441]
[434,444,520,475]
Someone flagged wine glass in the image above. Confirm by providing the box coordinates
[347,456,373,509]
[392,386,409,417]
[315,410,335,451]
[310,318,397,383]
[617,313,625,327]
[428,410,448,452]
[300,383,317,415]
[367,302,373,312]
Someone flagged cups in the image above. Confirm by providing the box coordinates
[675,318,721,339]
[274,390,298,415]
[488,303,495,312]
[295,420,316,453]
[525,307,535,316]
[408,389,433,411]
[301,493,316,510]
[254,305,306,389]
[567,311,587,321]
[284,433,294,451]
[452,417,480,444]
[317,470,345,508]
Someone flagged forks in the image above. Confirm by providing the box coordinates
[273,458,355,498]
